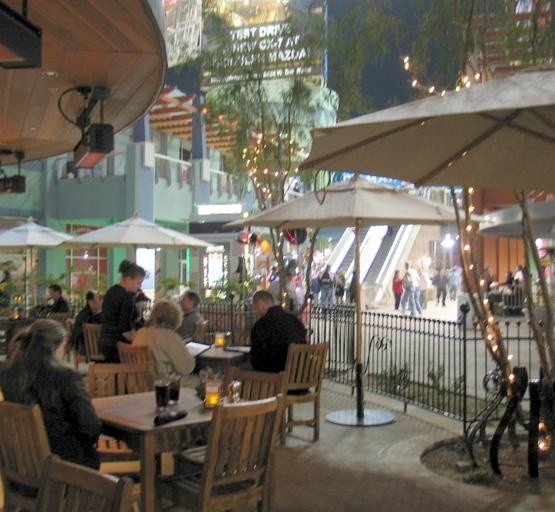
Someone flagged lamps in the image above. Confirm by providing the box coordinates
[0,0,42,69]
[0,149,25,193]
[57,85,113,169]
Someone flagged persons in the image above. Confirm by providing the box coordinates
[71,289,103,357]
[319,264,359,321]
[176,290,206,341]
[46,285,70,313]
[133,300,195,403]
[392,262,424,319]
[2,270,17,308]
[482,263,524,304]
[99,260,147,364]
[433,263,464,306]
[235,290,311,399]
[0,318,102,473]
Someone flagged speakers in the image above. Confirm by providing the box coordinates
[199,159,210,179]
[143,140,156,168]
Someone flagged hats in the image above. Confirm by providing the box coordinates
[88,289,104,299]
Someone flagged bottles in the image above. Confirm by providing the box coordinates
[227,380,241,404]
[144,301,154,316]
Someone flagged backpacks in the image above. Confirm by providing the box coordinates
[321,271,332,287]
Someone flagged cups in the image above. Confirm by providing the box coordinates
[205,383,219,407]
[152,375,179,410]
[214,332,231,348]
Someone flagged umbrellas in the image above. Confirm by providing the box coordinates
[222,174,495,417]
[297,62,555,193]
[59,210,210,265]
[0,215,73,308]
